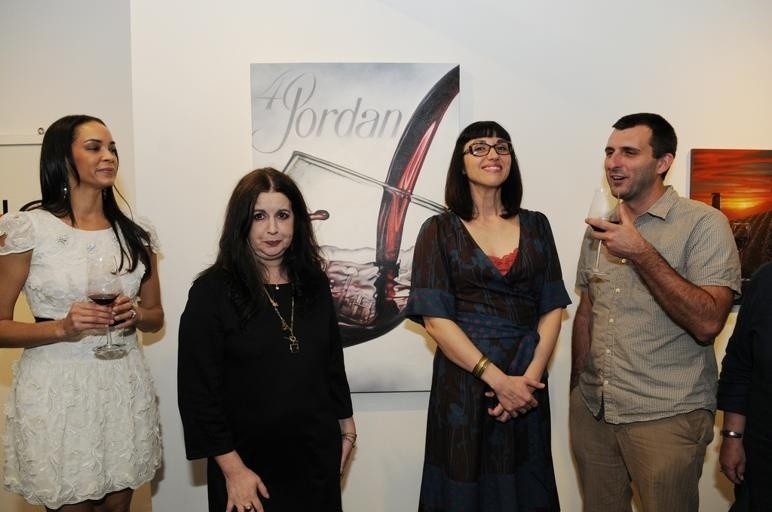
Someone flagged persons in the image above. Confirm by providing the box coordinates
[1,115,166,510]
[570,113,734,512]
[720,262,771,511]
[176,167,359,512]
[418,121,566,512]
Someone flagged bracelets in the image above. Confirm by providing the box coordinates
[338,431,358,449]
[465,352,497,382]
[719,429,743,440]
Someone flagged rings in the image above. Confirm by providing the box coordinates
[126,308,139,321]
[244,500,256,512]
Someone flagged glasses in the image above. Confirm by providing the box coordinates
[463,143,512,157]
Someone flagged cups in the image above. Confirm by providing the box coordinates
[279,149,452,348]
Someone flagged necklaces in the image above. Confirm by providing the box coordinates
[253,271,305,354]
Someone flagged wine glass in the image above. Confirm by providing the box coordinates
[580,186,622,278]
[84,252,126,356]
